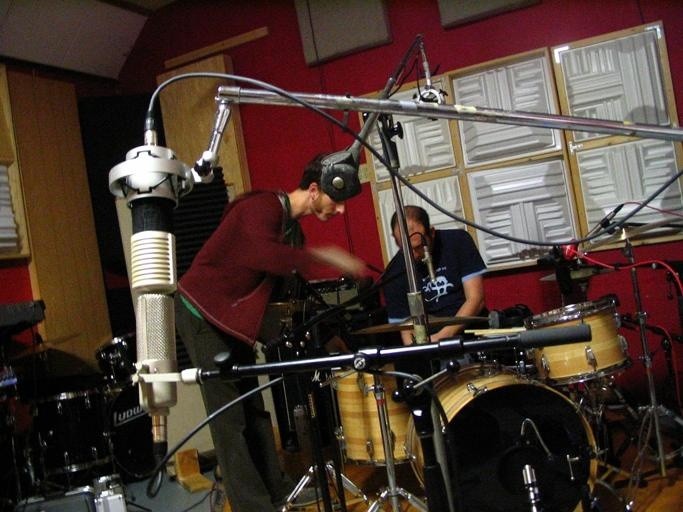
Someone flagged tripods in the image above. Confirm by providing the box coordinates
[369,368,429,511]
[614,239,683,477]
[280,373,369,512]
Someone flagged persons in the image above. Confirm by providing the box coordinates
[380,205,491,387]
[175,151,364,511]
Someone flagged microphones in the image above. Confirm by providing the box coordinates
[115,142,176,445]
[421,236,436,283]
[585,204,623,239]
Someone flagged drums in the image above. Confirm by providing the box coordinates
[330,364,414,466]
[406,362,600,512]
[94,331,137,393]
[525,300,633,386]
[24,392,115,478]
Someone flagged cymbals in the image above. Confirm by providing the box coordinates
[578,227,683,252]
[538,269,617,280]
[8,335,72,361]
[347,317,491,335]
